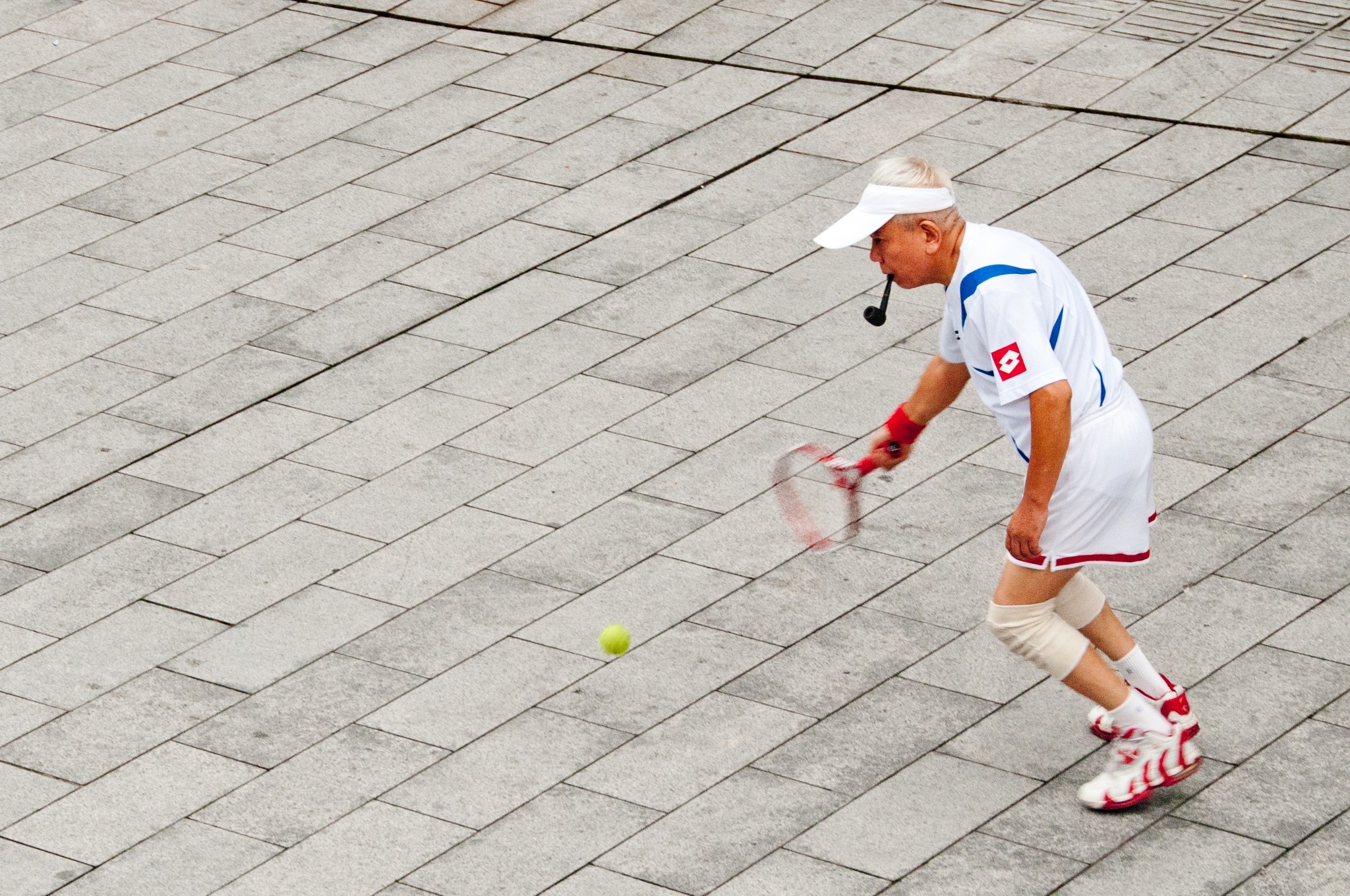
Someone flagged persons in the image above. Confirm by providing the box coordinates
[810,155,1204,811]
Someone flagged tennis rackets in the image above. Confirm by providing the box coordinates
[771,439,900,553]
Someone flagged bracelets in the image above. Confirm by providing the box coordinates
[885,402,924,447]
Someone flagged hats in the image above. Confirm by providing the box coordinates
[813,182,954,249]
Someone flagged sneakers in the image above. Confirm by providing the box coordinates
[1076,722,1203,811]
[1087,672,1200,744]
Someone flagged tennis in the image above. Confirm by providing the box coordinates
[599,625,631,656]
